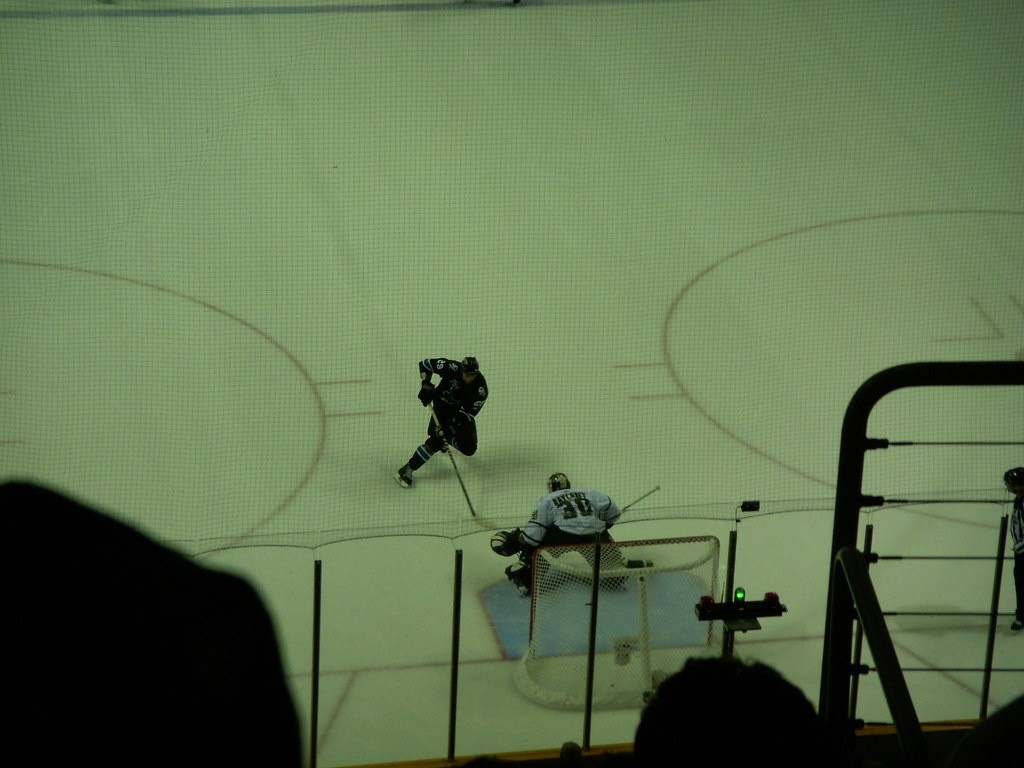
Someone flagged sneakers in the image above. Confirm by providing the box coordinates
[393,463,413,489]
[441,447,448,453]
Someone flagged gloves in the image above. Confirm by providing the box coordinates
[435,425,455,440]
[418,380,435,407]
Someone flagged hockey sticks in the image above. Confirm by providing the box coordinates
[428,402,476,516]
[620,485,661,511]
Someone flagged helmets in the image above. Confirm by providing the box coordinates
[461,356,478,374]
[548,473,570,492]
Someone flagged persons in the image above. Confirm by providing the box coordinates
[490,472,654,598]
[1003,467,1024,632]
[394,357,490,489]
[1,477,1024,768]
